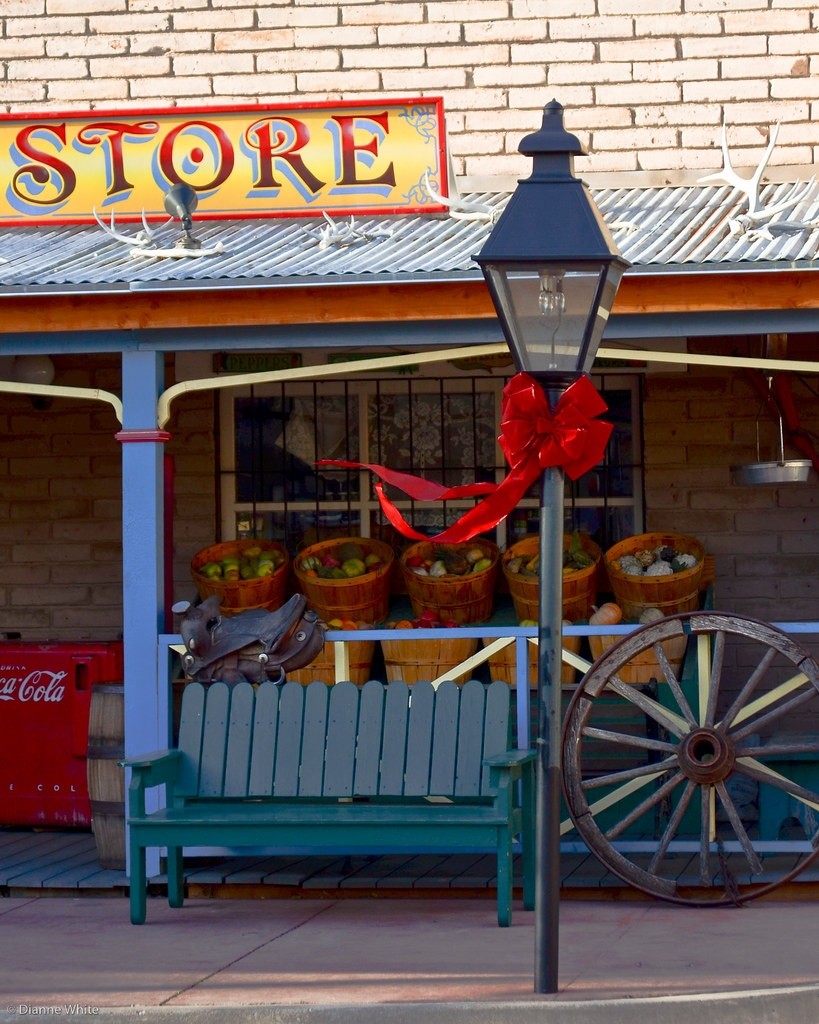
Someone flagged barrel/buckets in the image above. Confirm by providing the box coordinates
[378,637,480,687]
[400,533,501,626]
[281,635,377,689]
[293,537,396,627]
[482,636,581,687]
[190,537,291,623]
[502,531,604,623]
[86,681,128,869]
[604,532,705,623]
[586,623,688,684]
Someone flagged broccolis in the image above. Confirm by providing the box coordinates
[615,545,698,577]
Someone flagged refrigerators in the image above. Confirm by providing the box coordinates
[0,641,124,828]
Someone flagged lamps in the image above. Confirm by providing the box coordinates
[163,184,203,252]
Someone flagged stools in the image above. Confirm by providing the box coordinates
[753,754,819,857]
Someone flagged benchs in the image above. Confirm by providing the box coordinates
[115,680,536,928]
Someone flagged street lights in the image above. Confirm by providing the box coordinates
[471,97,631,994]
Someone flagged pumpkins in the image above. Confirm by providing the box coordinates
[300,540,665,629]
